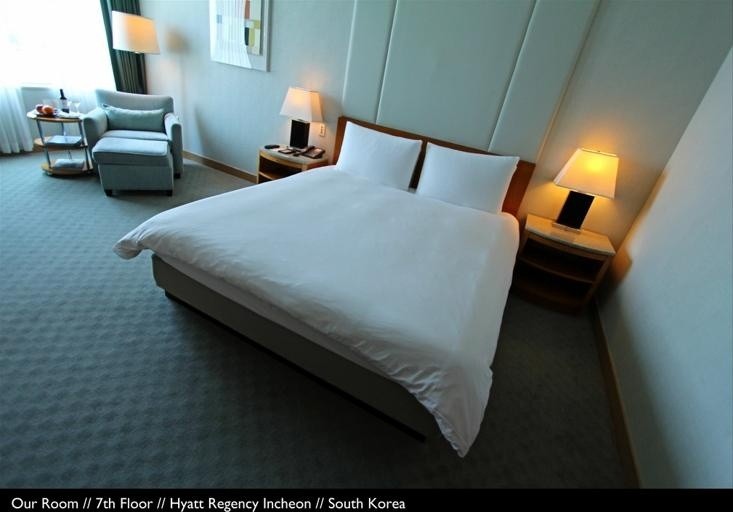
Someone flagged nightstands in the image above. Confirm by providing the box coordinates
[254,144,327,184]
[511,212,617,321]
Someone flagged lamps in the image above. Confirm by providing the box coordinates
[553,145,623,233]
[279,85,324,154]
[109,9,162,56]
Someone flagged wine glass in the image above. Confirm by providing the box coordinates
[74,103,82,116]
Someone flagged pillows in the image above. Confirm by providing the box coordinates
[336,117,521,219]
[101,102,166,131]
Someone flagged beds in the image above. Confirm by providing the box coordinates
[151,112,537,444]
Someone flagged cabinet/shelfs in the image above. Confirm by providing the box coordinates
[26,108,92,180]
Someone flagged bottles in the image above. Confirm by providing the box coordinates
[59,89,70,114]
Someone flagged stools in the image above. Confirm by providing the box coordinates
[90,136,177,197]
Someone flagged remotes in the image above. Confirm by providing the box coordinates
[264,144,280,148]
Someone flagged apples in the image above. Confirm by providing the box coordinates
[36,105,43,112]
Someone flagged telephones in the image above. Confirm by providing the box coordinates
[301,145,325,158]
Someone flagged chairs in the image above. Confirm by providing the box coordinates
[83,89,187,181]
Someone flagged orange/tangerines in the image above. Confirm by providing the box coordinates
[42,106,53,114]
